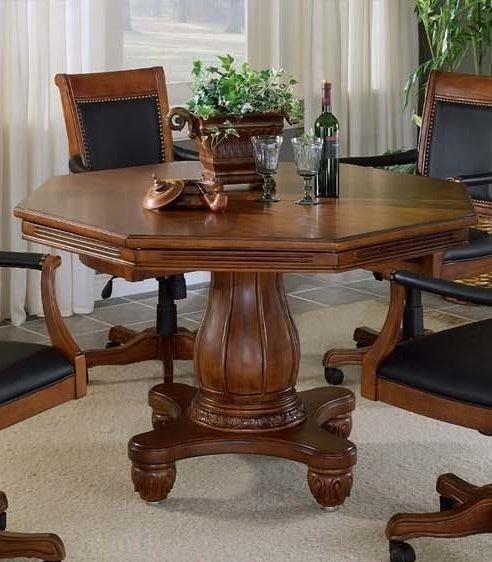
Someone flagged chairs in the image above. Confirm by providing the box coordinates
[319,71,492,384]
[0,251,86,562]
[54,65,197,386]
[361,260,492,561]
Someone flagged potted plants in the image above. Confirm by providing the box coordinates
[166,54,304,198]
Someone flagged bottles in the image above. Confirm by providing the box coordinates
[314,80,340,198]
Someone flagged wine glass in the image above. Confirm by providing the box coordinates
[251,134,324,205]
